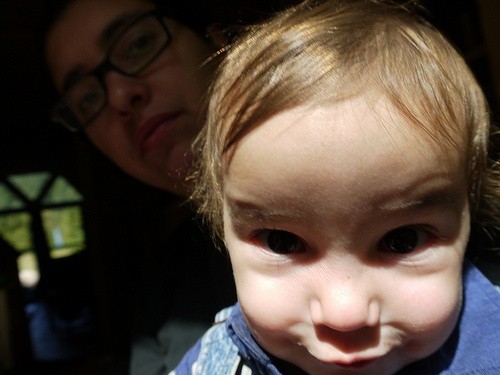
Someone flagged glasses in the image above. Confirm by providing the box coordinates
[46,10,172,131]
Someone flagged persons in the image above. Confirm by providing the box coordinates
[167,0,499,375]
[41,0,229,197]
